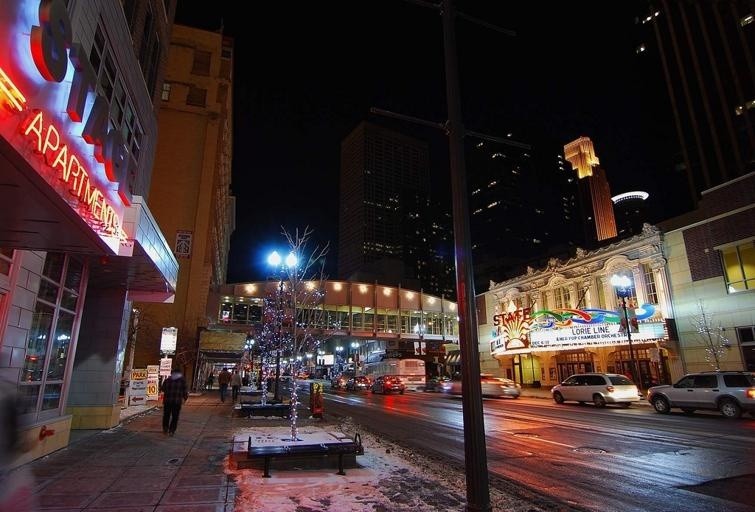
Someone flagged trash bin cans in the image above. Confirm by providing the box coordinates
[309,382,324,420]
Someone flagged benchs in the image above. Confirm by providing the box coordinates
[239,400,301,419]
[248,432,361,478]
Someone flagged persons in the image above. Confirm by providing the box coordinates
[242,373,275,394]
[207,370,215,391]
[160,365,189,436]
[230,371,235,387]
[230,370,242,403]
[218,367,231,403]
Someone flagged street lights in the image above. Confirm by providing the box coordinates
[243,339,254,363]
[270,340,359,376]
[607,273,639,386]
[413,322,425,358]
[268,249,297,402]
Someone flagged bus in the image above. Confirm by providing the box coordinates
[364,358,426,392]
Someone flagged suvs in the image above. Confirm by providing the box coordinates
[647,370,755,421]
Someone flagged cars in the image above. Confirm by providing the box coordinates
[369,375,407,394]
[426,375,453,393]
[331,373,352,389]
[452,373,522,400]
[345,376,369,392]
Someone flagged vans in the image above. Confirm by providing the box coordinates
[550,371,641,409]
